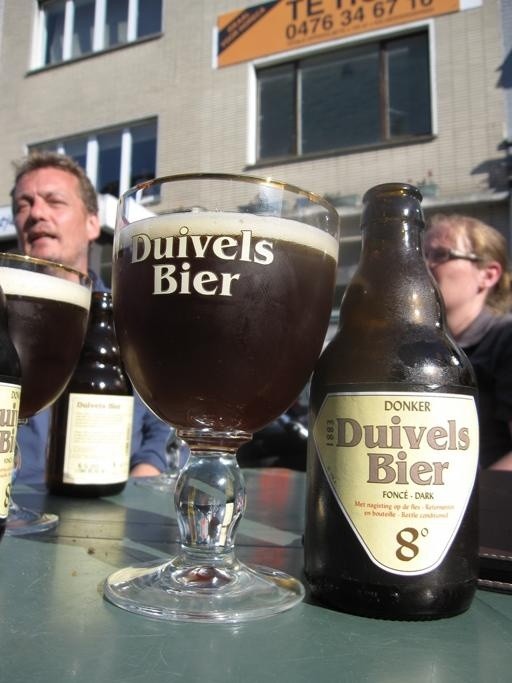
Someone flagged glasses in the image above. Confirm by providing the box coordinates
[422,243,487,264]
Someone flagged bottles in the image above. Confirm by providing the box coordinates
[276,414,308,443]
[304,184,480,621]
[0,283,22,540]
[48,290,135,497]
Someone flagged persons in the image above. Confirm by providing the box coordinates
[10,154,173,484]
[419,213,511,472]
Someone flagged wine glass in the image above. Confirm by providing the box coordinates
[0,250,92,536]
[102,174,342,623]
